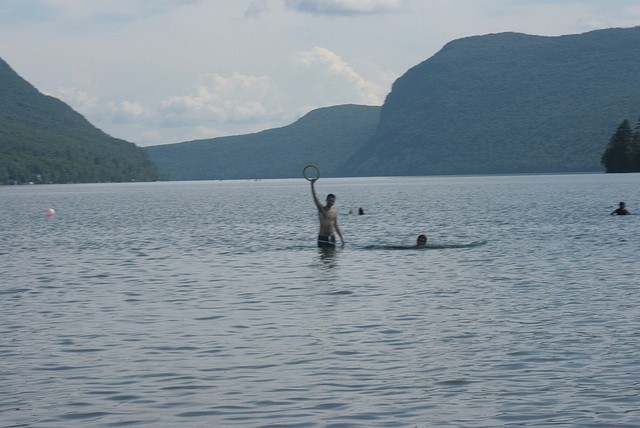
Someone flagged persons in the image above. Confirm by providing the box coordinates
[311,180,344,249]
[348,206,367,218]
[610,202,632,215]
[417,234,427,245]
